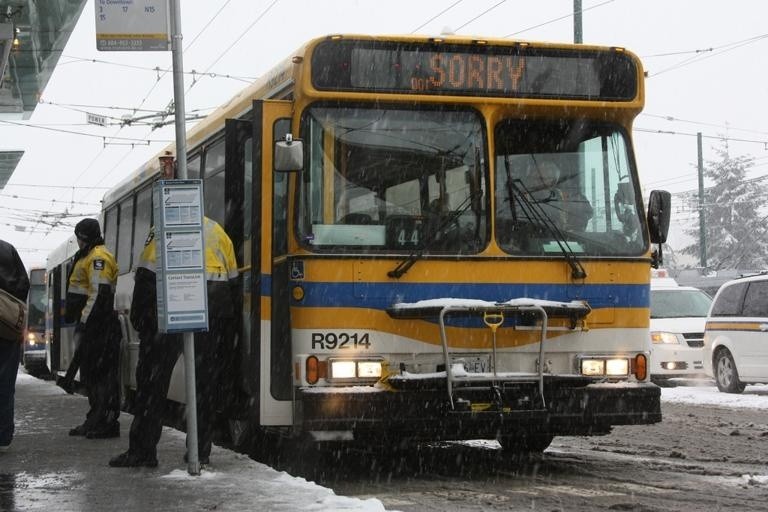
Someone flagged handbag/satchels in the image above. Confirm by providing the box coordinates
[0,289,28,342]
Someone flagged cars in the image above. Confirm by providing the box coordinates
[649,286,713,379]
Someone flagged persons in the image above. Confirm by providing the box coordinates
[0,238,31,450]
[108,214,241,467]
[63,218,123,440]
[517,159,568,232]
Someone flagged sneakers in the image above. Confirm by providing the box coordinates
[184,451,209,464]
[69,420,120,438]
[0,440,12,452]
[109,448,157,467]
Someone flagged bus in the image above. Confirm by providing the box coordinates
[21,210,98,383]
[100,34,671,452]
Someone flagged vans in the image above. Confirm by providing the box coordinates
[702,271,768,393]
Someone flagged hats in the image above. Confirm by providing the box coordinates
[75,219,100,242]
